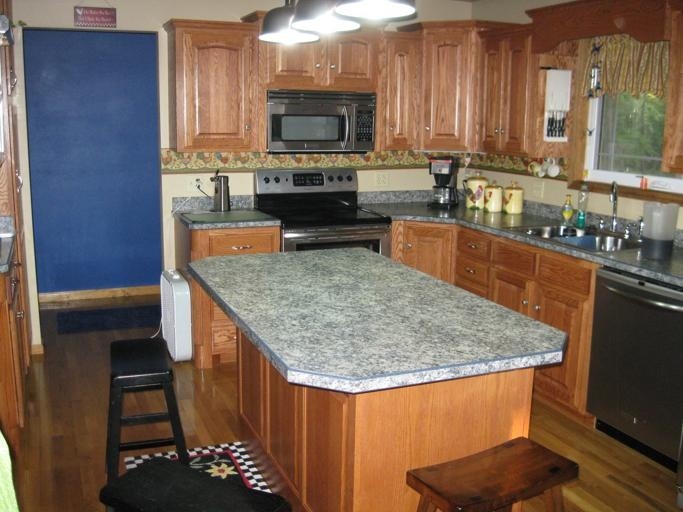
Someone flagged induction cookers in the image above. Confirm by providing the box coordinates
[254,191,390,224]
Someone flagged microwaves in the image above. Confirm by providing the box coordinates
[261,84,376,154]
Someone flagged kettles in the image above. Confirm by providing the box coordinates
[209,174,230,212]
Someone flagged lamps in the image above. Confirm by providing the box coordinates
[252,1,420,49]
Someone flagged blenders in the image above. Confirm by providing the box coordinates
[425,153,459,212]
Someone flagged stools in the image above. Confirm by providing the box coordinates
[102,333,191,483]
[98,454,290,512]
[404,432,586,512]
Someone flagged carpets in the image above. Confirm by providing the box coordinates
[55,302,162,336]
[118,437,275,496]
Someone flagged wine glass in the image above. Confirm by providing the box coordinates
[546,109,567,137]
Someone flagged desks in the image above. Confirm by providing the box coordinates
[181,244,569,512]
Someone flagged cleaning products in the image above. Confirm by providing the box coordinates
[559,194,573,227]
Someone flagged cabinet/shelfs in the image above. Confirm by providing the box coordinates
[0,260,26,458]
[418,19,485,156]
[162,16,267,156]
[0,0,33,382]
[258,20,378,96]
[655,2,683,178]
[172,220,281,372]
[367,29,420,155]
[388,204,598,424]
[476,25,580,164]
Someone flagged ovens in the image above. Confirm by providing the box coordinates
[280,220,393,263]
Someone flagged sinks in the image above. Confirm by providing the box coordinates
[500,224,597,240]
[549,231,644,253]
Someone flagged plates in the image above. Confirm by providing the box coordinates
[548,164,559,178]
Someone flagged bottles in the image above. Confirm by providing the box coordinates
[558,191,574,226]
[461,170,525,215]
[575,168,588,230]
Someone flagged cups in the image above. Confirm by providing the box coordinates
[640,196,679,266]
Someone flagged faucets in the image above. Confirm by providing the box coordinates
[609,181,618,233]
